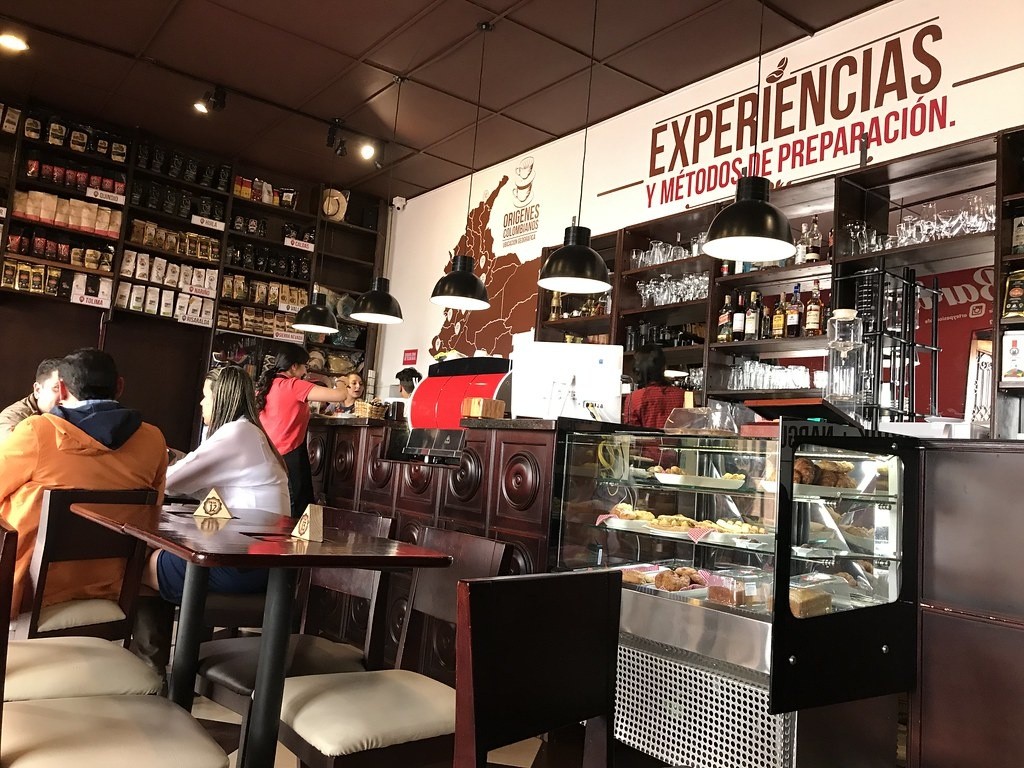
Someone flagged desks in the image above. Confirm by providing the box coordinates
[70,501,342,714]
[124,523,453,767]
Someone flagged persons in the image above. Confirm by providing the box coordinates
[326,372,366,413]
[130,366,291,699]
[0,357,66,433]
[254,343,350,523]
[395,367,422,398]
[0,347,169,621]
[624,343,686,555]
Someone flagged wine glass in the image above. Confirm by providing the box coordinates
[844,194,996,256]
[630,232,709,307]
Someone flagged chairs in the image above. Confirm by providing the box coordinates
[0,444,622,768]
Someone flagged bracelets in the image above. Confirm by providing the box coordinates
[335,380,346,387]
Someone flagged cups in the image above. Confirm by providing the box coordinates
[727,360,828,391]
[391,401,404,421]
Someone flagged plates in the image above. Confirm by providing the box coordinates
[615,562,734,597]
[760,480,862,499]
[654,473,745,490]
[602,517,775,547]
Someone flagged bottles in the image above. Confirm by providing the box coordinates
[716,214,831,344]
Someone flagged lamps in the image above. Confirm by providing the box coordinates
[325,124,347,157]
[537,0,612,294]
[194,89,228,113]
[350,76,405,324]
[293,120,340,334]
[429,21,493,310]
[701,3,796,263]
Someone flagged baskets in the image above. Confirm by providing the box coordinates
[354,400,388,420]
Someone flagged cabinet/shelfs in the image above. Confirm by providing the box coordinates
[0,125,384,455]
[536,126,1024,437]
[559,432,921,768]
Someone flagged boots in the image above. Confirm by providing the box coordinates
[199,624,213,643]
[128,594,175,698]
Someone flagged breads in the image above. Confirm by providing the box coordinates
[607,458,856,618]
